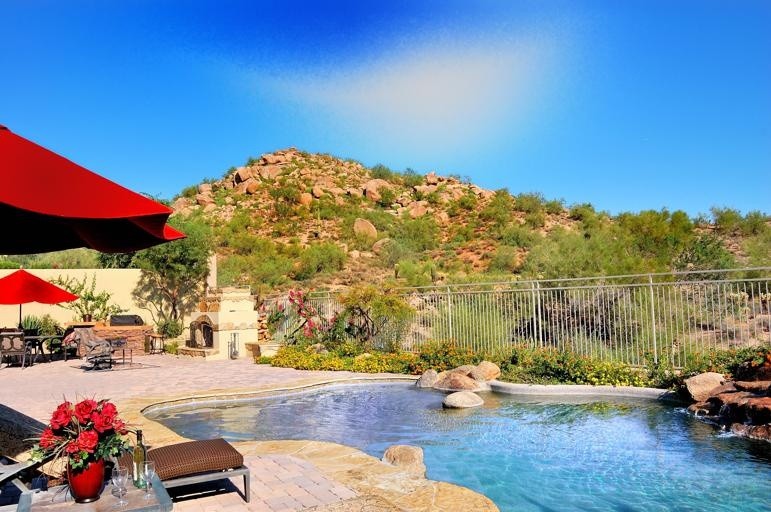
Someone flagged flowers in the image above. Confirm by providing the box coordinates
[23,394,142,503]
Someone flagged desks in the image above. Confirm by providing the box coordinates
[146,332,168,355]
[18,471,173,512]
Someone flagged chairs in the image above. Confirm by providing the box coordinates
[2,327,135,371]
[102,437,251,502]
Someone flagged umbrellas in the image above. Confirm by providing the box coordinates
[0,265,79,328]
[0,121,188,255]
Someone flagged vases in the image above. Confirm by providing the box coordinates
[66,455,105,504]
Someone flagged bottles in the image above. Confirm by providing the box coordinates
[133,429,147,486]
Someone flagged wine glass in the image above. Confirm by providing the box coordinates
[112,466,129,508]
[139,461,155,500]
